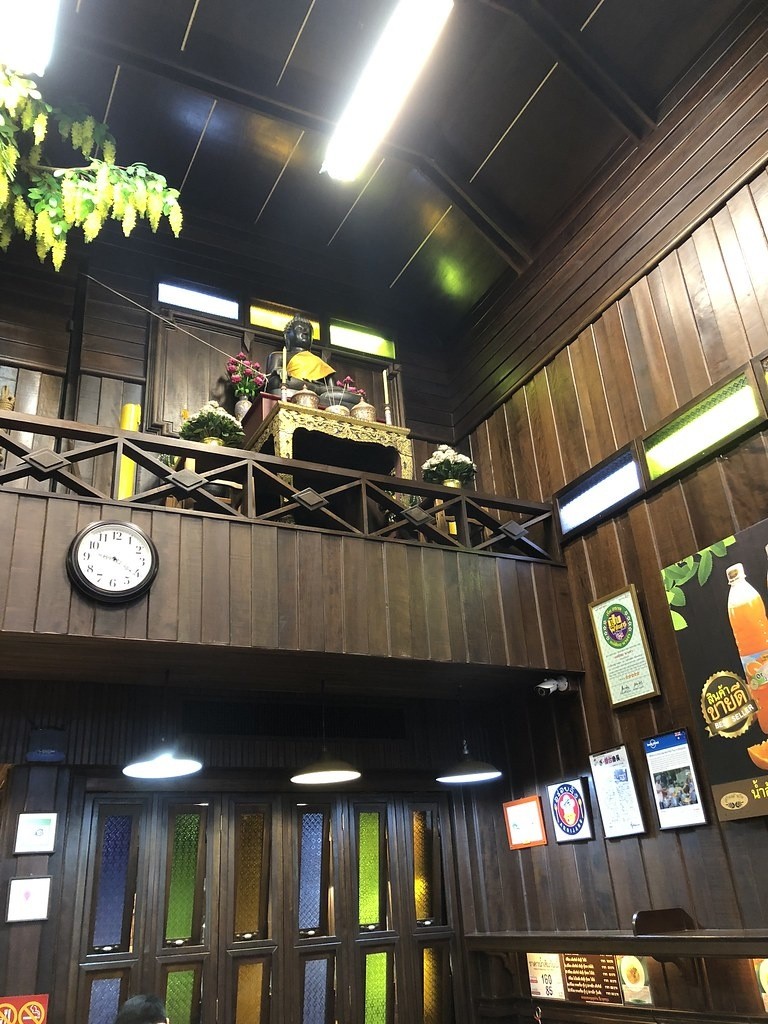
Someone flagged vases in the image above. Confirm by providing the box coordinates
[442,479,461,491]
[204,435,225,449]
[234,391,253,422]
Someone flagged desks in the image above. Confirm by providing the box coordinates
[246,403,417,535]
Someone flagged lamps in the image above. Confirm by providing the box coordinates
[121,663,202,778]
[289,671,362,784]
[436,680,502,792]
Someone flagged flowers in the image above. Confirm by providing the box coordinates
[422,444,477,487]
[177,399,246,448]
[228,351,267,396]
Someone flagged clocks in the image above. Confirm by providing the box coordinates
[65,520,159,603]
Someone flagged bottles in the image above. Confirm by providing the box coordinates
[292,385,320,409]
[351,396,376,423]
[726,563,768,733]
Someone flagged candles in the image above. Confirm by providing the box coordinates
[382,370,389,405]
[282,346,287,384]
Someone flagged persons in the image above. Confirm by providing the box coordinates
[117,995,170,1024]
[265,317,366,403]
